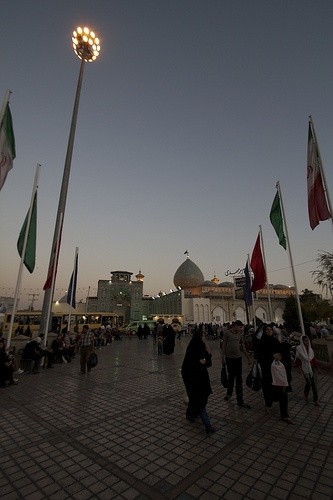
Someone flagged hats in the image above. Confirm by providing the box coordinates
[83,325,89,330]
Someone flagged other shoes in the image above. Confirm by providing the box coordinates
[315,401,323,406]
[239,402,250,408]
[224,396,229,400]
[205,427,216,435]
[282,416,292,424]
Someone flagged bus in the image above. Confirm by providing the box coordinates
[2,311,121,339]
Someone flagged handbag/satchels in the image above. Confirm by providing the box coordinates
[271,360,289,386]
[220,364,228,388]
[246,362,262,391]
[89,353,98,367]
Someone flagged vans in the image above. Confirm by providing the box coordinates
[126,320,157,335]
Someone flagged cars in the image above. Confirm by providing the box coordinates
[117,324,129,333]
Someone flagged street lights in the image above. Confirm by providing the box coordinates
[36,26,101,357]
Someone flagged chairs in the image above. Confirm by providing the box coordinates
[16,348,26,372]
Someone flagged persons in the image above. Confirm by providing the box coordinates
[137,322,150,340]
[0,332,54,387]
[253,323,297,425]
[220,320,250,409]
[51,324,123,364]
[294,335,324,406]
[180,331,218,437]
[78,325,91,375]
[175,319,332,341]
[0,313,131,338]
[151,318,176,359]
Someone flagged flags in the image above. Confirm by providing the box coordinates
[0,99,16,191]
[243,231,268,307]
[66,252,78,308]
[269,188,287,251]
[41,220,61,292]
[184,251,187,254]
[16,187,38,274]
[305,119,332,231]
